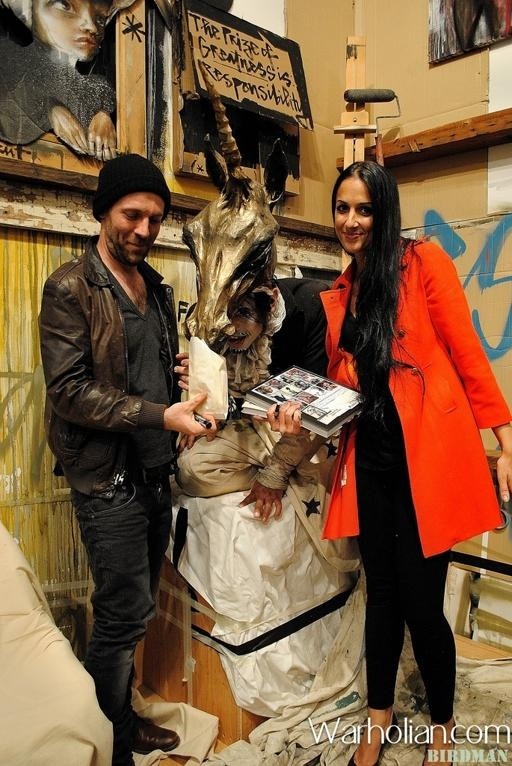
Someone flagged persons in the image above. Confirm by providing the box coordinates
[0,0,120,165]
[319,159,511,766]
[171,273,340,526]
[35,153,219,766]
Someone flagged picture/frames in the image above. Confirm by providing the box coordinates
[0,0,146,189]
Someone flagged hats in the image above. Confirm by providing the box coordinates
[93,154,170,222]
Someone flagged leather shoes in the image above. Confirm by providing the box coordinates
[131,716,180,754]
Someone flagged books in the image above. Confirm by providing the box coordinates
[244,365,366,432]
[242,401,357,438]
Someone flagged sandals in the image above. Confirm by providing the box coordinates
[422,719,456,766]
[347,713,397,766]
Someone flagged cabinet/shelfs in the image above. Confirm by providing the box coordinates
[133,490,360,747]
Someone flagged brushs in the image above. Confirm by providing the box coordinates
[344,90,402,167]
